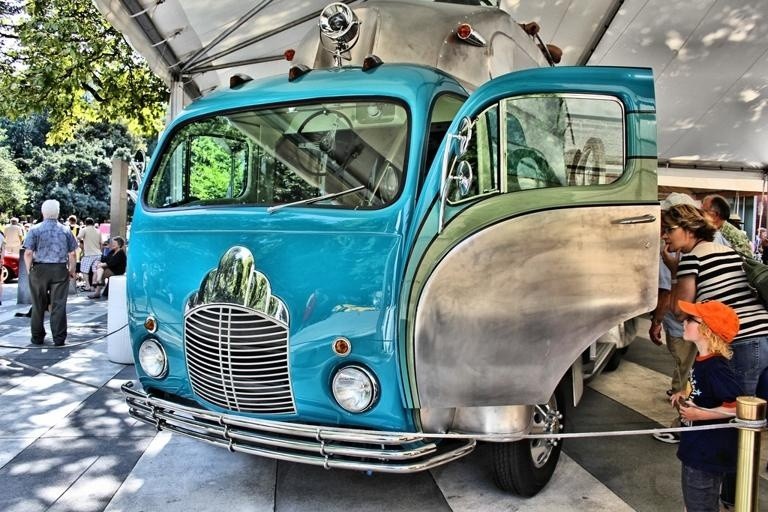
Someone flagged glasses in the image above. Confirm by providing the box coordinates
[686,316,701,325]
[662,224,679,233]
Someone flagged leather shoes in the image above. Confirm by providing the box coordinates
[81,280,104,298]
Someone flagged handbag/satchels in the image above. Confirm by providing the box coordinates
[735,249,768,311]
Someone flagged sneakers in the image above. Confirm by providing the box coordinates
[652,427,681,443]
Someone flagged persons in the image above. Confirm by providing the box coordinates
[648,190,768,512]
[0,199,127,346]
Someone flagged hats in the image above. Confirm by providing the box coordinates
[679,299,740,343]
[661,192,701,211]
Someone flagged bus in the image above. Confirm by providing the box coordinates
[121,0,662,499]
